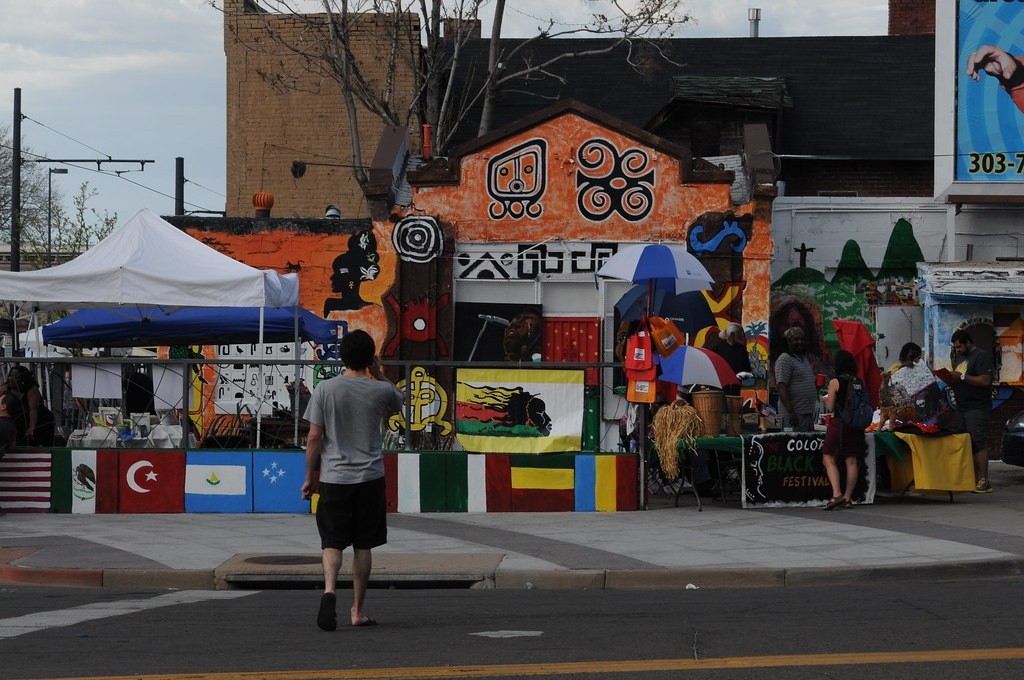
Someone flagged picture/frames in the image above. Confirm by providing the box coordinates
[130,412,150,438]
[98,407,124,427]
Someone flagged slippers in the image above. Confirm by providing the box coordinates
[358,614,376,626]
[317,592,336,631]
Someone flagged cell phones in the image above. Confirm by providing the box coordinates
[367,355,375,366]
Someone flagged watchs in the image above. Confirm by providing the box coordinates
[960,373,965,382]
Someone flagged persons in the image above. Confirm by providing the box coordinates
[0,366,45,447]
[774,326,818,432]
[887,342,922,378]
[966,45,1024,114]
[700,322,751,395]
[948,330,993,492]
[299,329,408,632]
[822,350,865,510]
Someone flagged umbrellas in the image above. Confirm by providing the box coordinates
[596,243,715,315]
[614,285,711,330]
[657,332,743,390]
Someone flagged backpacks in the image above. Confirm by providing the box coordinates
[834,374,873,430]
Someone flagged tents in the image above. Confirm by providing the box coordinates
[0,207,301,450]
[43,304,338,449]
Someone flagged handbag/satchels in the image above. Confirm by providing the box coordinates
[625,315,686,402]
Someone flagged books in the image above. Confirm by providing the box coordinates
[929,368,953,387]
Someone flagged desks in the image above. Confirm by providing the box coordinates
[645,431,977,513]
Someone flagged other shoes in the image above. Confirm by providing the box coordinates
[972,478,992,493]
[822,495,843,510]
[841,500,852,508]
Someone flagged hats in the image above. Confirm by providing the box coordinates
[784,327,804,341]
[834,349,855,374]
[727,323,740,336]
